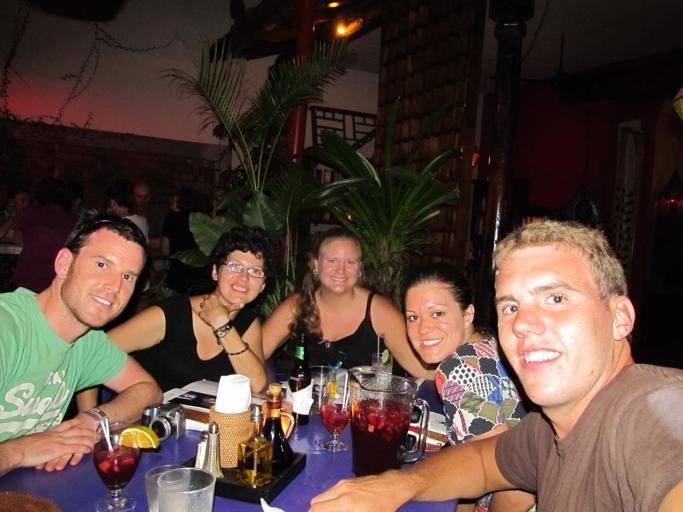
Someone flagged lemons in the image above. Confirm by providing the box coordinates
[117,424,159,449]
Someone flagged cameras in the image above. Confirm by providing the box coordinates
[141,403,187,442]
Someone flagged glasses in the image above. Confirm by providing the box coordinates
[215,259,266,279]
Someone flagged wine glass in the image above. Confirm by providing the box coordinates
[316,368,351,453]
[94,423,141,512]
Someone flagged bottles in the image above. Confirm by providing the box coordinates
[262,380,295,476]
[194,422,225,478]
[288,330,312,425]
[237,404,274,489]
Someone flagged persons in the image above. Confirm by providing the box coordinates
[261,227,438,382]
[403,262,538,512]
[308,218,682,512]
[0,174,206,292]
[0,211,163,477]
[76,225,277,415]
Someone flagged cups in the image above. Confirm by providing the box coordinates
[308,366,335,416]
[144,464,215,512]
[349,366,430,478]
[370,354,396,382]
[261,400,295,441]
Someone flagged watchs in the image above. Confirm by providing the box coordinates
[214,320,234,338]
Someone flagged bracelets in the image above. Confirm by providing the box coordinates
[82,407,109,434]
[225,343,249,355]
